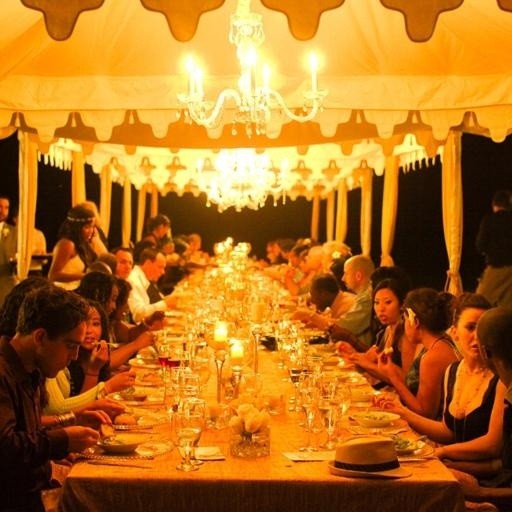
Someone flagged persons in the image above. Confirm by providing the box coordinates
[447,305,512,499]
[380,293,508,462]
[258,237,417,385]
[1,201,217,511]
[378,288,460,418]
[477,190,511,304]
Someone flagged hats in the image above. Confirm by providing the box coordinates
[327,433,414,479]
[64,201,100,227]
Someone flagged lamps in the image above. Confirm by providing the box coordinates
[189,149,291,211]
[173,1,328,138]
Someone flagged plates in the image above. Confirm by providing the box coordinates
[127,358,160,369]
[98,434,147,455]
[348,410,401,427]
[394,438,422,454]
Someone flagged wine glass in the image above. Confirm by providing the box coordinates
[158,336,215,472]
[277,349,352,454]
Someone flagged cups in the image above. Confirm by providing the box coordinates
[265,393,285,416]
[210,404,229,429]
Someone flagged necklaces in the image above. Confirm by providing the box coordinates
[456,366,490,414]
[427,336,442,354]
